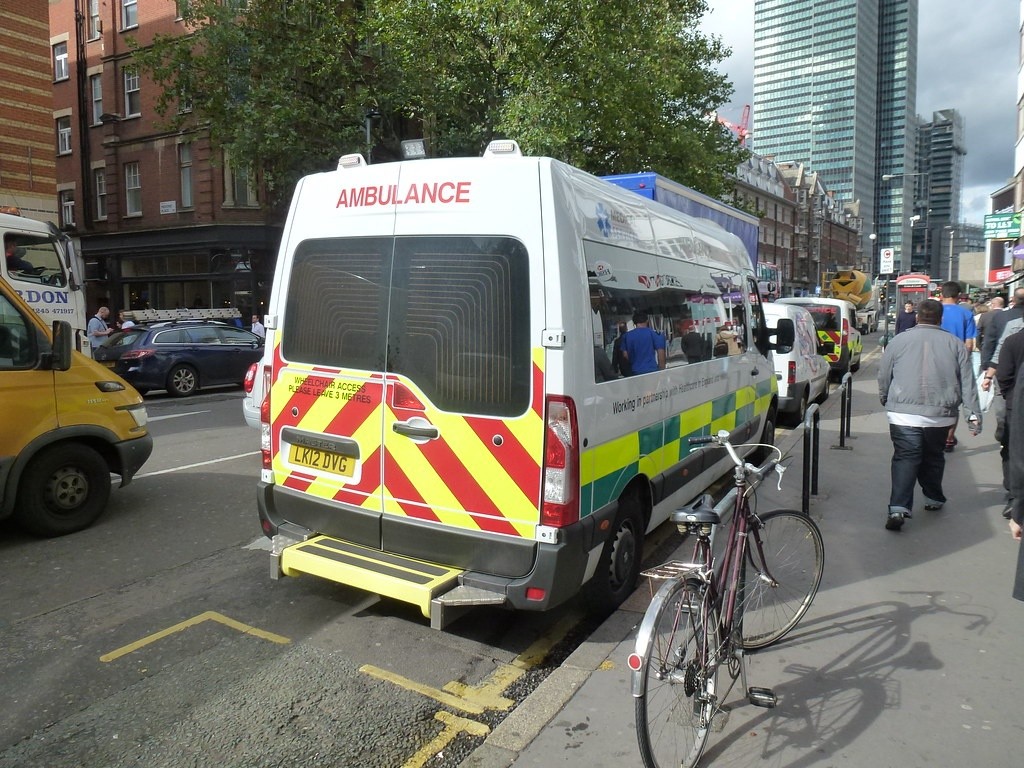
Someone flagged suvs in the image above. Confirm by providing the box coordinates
[93,319,266,398]
[762,303,831,423]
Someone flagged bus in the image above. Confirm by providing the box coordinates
[595,172,759,276]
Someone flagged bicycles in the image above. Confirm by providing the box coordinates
[627,430,825,768]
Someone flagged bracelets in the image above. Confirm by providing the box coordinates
[984,375,993,382]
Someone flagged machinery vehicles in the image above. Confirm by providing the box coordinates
[831,267,880,335]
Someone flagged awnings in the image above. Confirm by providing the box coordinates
[990,272,1024,286]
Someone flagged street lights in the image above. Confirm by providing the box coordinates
[883,170,932,276]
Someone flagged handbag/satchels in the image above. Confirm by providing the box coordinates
[975,371,994,414]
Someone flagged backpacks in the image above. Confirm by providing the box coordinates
[714,334,728,357]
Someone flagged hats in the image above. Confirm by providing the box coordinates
[687,325,696,333]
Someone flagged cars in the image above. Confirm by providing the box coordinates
[888,306,896,323]
[242,351,265,431]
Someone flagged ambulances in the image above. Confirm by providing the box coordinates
[776,297,862,379]
[258,137,794,634]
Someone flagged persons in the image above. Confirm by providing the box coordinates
[87,307,114,351]
[117,310,135,329]
[612,321,631,377]
[621,309,666,374]
[940,281,976,449]
[680,324,703,364]
[6,239,27,270]
[718,319,747,356]
[594,344,618,382]
[973,286,1024,601]
[877,299,982,531]
[895,300,917,335]
[251,314,266,337]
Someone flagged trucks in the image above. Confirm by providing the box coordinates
[0,208,91,358]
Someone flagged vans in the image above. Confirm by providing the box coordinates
[0,276,154,534]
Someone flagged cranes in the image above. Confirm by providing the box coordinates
[707,105,753,150]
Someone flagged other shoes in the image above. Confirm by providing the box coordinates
[924,503,942,511]
[1002,498,1012,516]
[945,438,958,452]
[885,513,904,530]
